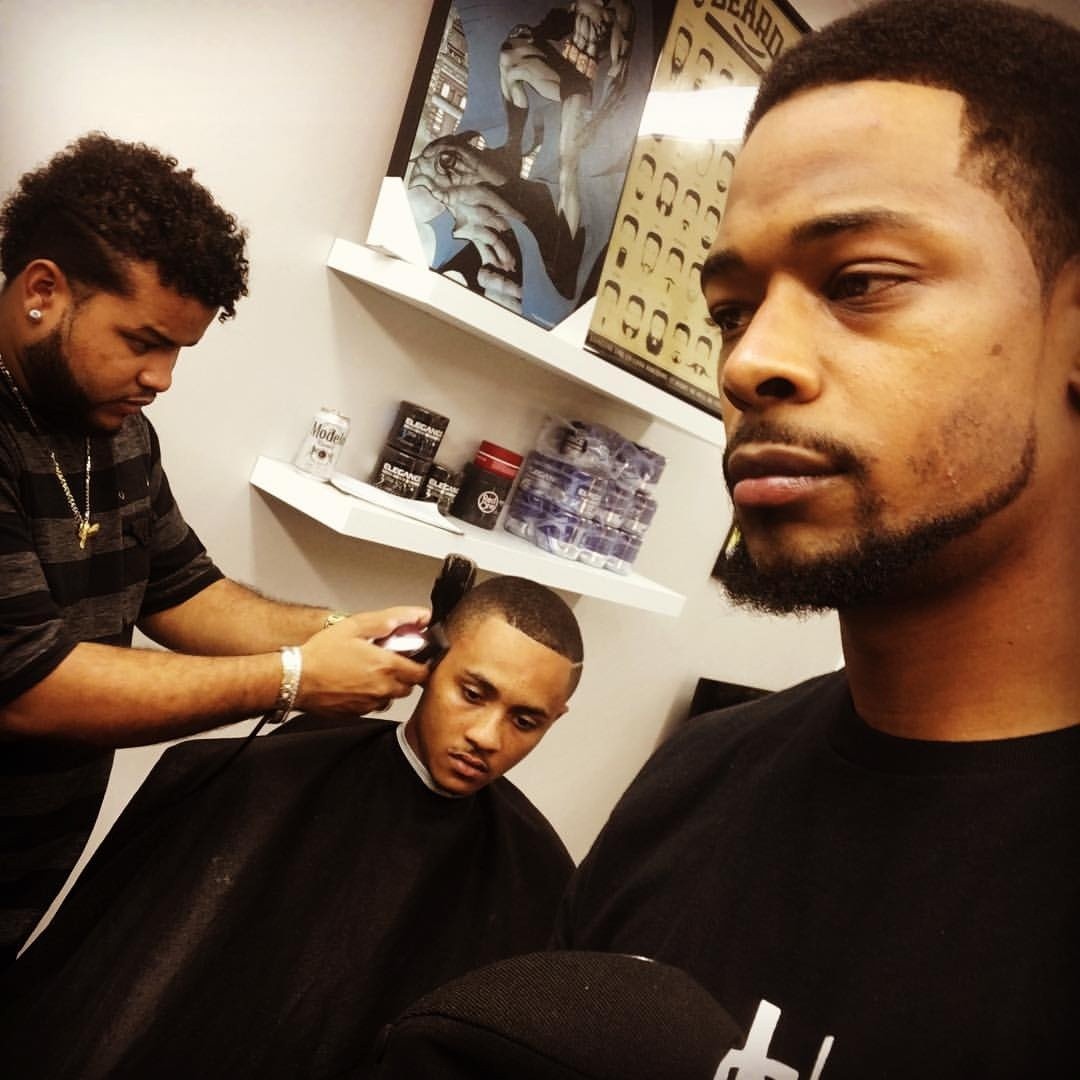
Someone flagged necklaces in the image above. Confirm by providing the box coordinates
[0,354,99,549]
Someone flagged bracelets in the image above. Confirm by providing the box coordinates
[272,645,301,723]
[326,612,352,630]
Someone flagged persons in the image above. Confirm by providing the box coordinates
[558,0,1080,1080]
[0,132,426,964]
[0,576,586,1080]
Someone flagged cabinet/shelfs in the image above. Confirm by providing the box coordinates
[247,238,735,616]
